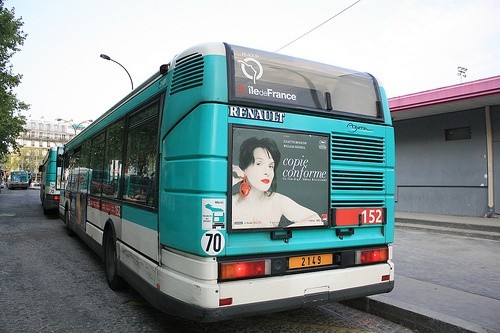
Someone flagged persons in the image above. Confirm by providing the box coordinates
[232,138,324,229]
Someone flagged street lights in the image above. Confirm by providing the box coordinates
[100,52,133,99]
[57,117,94,137]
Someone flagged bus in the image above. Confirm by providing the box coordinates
[59,42,397,325]
[39,146,63,218]
[7,170,29,189]
